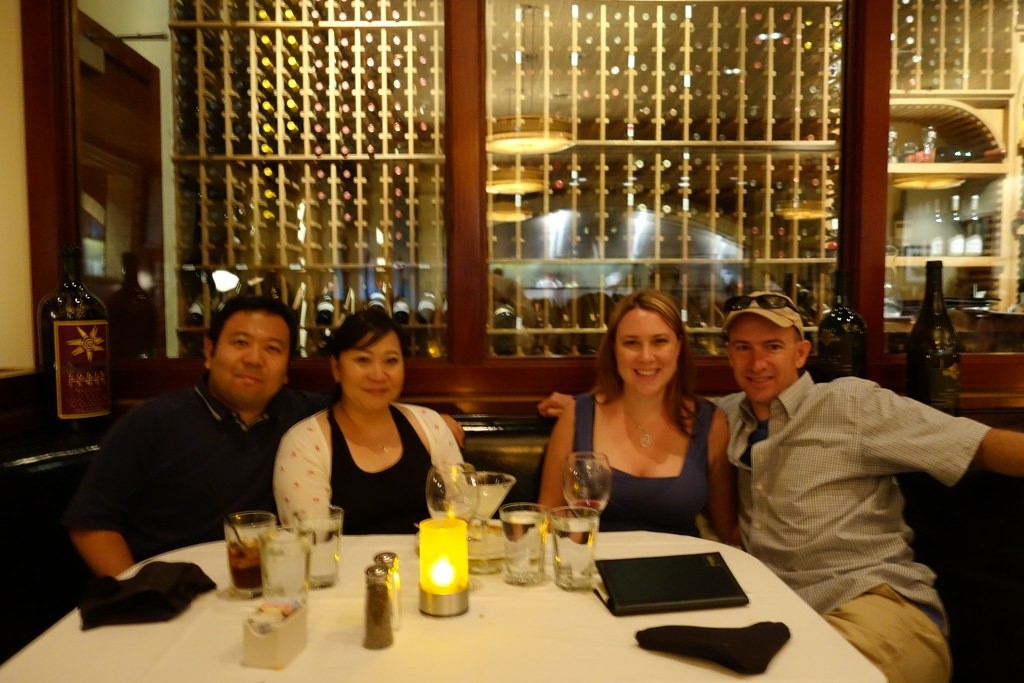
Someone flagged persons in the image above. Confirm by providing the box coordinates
[532,289,738,546]
[537,291,1024,683]
[64,293,461,583]
[273,309,460,535]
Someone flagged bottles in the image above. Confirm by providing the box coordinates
[818,268,865,381]
[183,0,1024,359]
[106,251,156,400]
[909,262,962,416]
[35,244,113,443]
[362,553,402,648]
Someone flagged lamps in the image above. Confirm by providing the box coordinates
[492,201,533,222]
[776,199,835,221]
[488,160,552,195]
[893,156,966,189]
[484,10,576,154]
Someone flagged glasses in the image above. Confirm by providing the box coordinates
[722,294,801,311]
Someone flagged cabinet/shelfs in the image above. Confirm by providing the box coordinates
[886,87,1016,309]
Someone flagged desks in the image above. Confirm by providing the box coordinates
[0,532,889,683]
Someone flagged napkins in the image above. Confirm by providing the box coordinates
[72,559,217,637]
[635,618,790,675]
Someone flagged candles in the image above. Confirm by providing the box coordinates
[419,516,468,617]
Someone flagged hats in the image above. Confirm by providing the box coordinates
[722,291,804,340]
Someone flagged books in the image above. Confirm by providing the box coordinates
[594,551,748,616]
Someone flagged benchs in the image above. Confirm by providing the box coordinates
[0,426,1024,683]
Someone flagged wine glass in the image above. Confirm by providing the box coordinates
[471,470,517,550]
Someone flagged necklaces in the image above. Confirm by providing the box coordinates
[628,409,661,447]
[377,442,388,453]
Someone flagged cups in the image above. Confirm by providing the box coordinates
[500,502,599,591]
[223,511,276,596]
[561,451,613,523]
[426,463,480,524]
[290,506,345,588]
[258,522,307,601]
[418,519,470,615]
